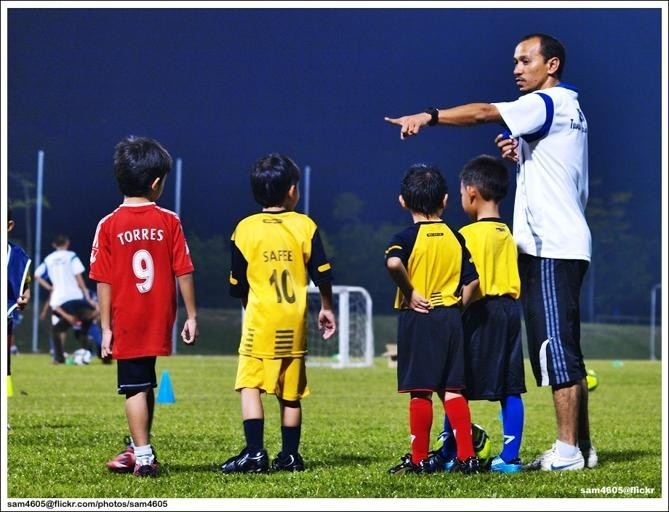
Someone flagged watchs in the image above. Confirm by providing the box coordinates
[423,106,439,127]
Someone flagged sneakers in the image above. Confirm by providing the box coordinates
[428,445,457,472]
[449,457,480,473]
[219,447,269,474]
[580,447,599,469]
[271,451,305,473]
[523,444,585,473]
[479,453,522,473]
[107,437,136,473]
[132,448,156,476]
[386,453,432,476]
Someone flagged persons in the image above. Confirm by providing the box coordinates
[7,207,31,397]
[34,233,114,365]
[385,163,484,473]
[89,137,199,478]
[416,154,527,473]
[384,34,598,471]
[220,153,337,474]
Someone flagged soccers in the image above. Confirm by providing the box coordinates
[72,348,93,366]
